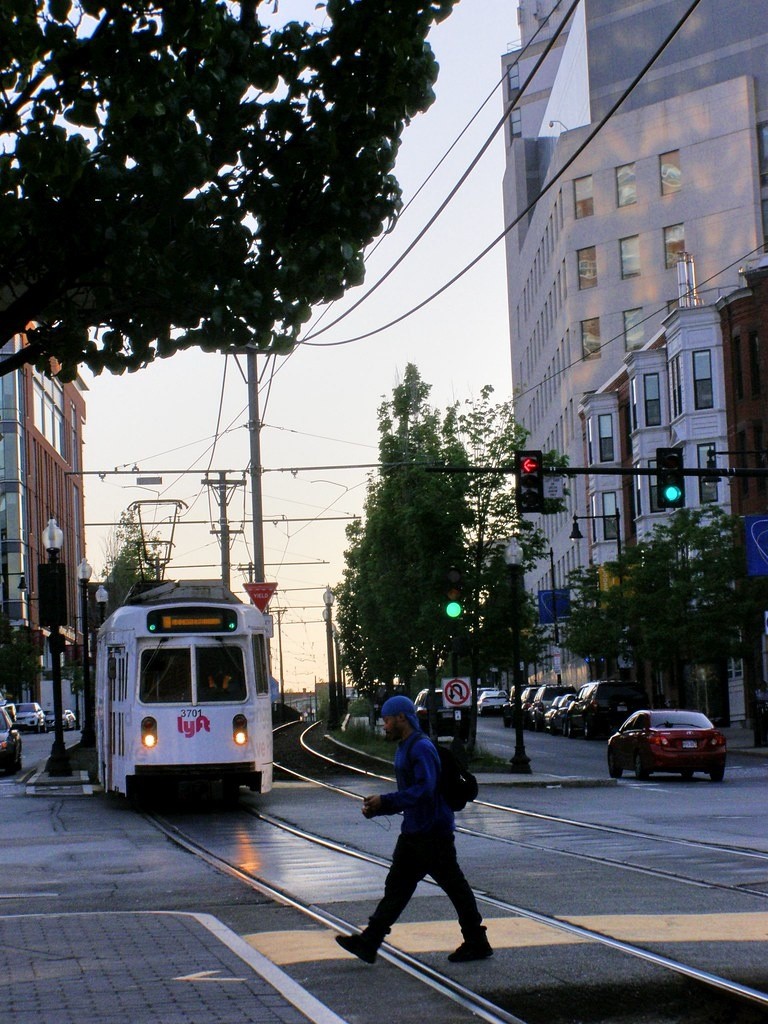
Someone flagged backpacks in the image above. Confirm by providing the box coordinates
[406,736,478,810]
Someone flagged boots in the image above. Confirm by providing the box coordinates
[447,926,492,963]
[336,916,392,964]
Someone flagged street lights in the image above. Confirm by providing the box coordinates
[41,514,73,778]
[95,584,109,627]
[502,538,533,775]
[322,590,340,731]
[330,622,346,716]
[75,558,95,748]
[568,508,630,682]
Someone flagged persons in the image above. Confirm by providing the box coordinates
[204,662,238,700]
[336,698,494,964]
[754,681,768,747]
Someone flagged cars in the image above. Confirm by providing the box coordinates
[476,690,509,718]
[0,693,23,777]
[607,709,726,781]
[11,702,46,734]
[542,693,578,736]
[43,710,78,731]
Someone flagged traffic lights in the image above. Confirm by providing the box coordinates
[442,564,463,620]
[515,449,543,513]
[656,447,686,507]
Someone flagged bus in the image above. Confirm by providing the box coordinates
[92,499,275,813]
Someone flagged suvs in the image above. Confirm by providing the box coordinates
[527,683,577,733]
[501,685,542,731]
[413,688,473,744]
[477,687,500,699]
[563,681,651,741]
